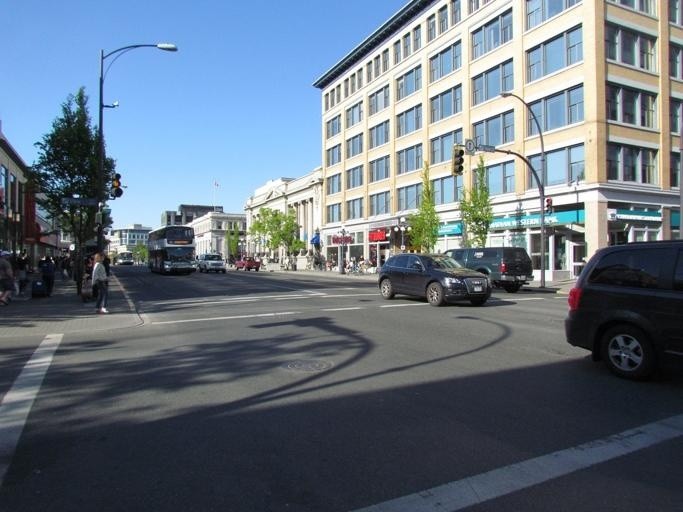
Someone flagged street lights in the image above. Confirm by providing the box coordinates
[336,224,349,273]
[237,237,246,258]
[498,91,546,288]
[94,43,177,281]
[393,217,412,253]
[478,144,495,153]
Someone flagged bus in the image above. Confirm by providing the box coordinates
[146,224,197,275]
[114,251,133,265]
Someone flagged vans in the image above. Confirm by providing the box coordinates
[443,247,533,294]
[563,238,683,379]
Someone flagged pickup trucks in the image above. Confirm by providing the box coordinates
[234,256,259,272]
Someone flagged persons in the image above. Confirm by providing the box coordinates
[0,250,14,306]
[86,256,93,280]
[16,251,28,296]
[50,256,55,265]
[89,251,110,313]
[40,256,55,298]
[102,254,111,277]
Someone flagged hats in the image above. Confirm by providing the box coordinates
[1,251,12,257]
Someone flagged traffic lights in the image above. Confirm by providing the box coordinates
[111,174,123,198]
[545,199,551,214]
[547,226,554,236]
[454,149,464,173]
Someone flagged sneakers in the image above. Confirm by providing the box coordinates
[97,310,109,315]
[0,295,9,306]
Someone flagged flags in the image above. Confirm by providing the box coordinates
[214,180,220,189]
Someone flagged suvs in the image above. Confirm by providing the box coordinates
[198,253,225,274]
[377,252,491,309]
